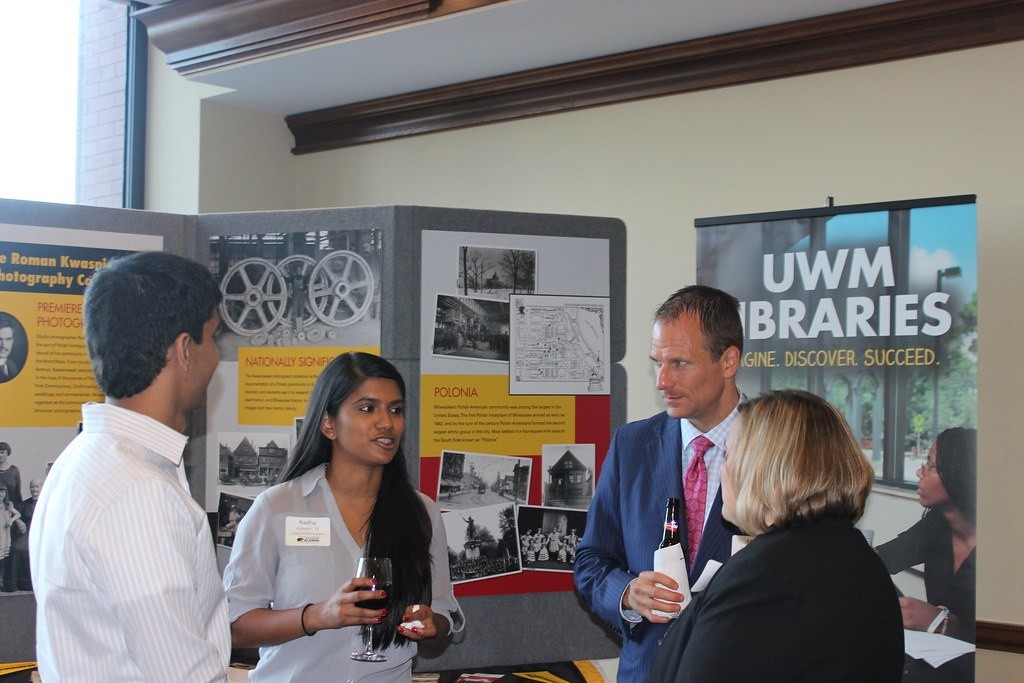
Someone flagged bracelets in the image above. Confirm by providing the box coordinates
[301,602,317,636]
[926,605,948,635]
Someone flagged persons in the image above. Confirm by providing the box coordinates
[222,351,465,683]
[0,317,18,383]
[874,426,977,645]
[571,284,757,683]
[26,247,233,683]
[450,556,515,579]
[648,388,904,683]
[281,265,320,330]
[441,323,489,351]
[230,505,242,532]
[521,528,583,563]
[0,442,43,592]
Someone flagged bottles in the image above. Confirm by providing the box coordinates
[658,497,680,550]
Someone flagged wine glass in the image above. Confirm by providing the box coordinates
[350,556,393,662]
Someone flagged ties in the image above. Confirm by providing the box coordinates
[683,436,716,573]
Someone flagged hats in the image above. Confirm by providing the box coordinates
[571,529,576,532]
[231,505,237,510]
[537,528,542,531]
[553,527,557,530]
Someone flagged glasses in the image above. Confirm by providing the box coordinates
[921,455,937,472]
